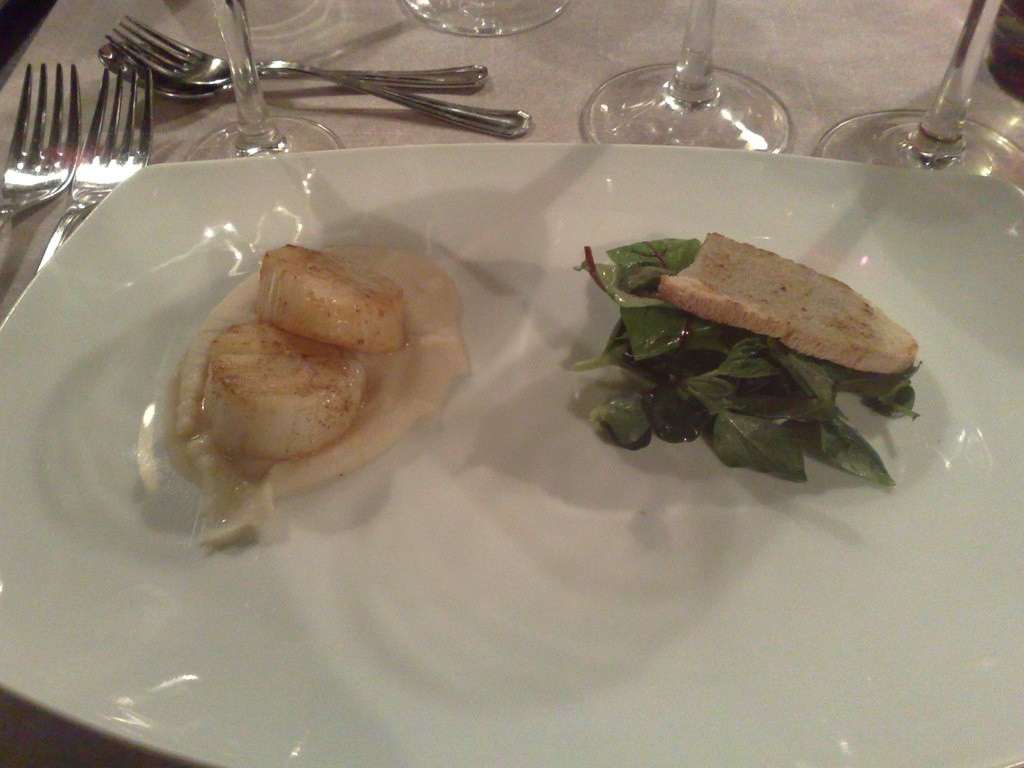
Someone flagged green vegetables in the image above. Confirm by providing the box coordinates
[565,237,921,487]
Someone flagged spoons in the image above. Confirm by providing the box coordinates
[98,41,488,98]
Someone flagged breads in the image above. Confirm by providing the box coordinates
[659,232,920,374]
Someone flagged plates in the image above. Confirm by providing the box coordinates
[0,142,1024,768]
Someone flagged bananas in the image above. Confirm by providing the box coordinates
[203,322,367,462]
[256,244,404,349]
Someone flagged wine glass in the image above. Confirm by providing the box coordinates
[406,0,570,37]
[182,0,347,161]
[812,0,1023,191]
[581,0,796,155]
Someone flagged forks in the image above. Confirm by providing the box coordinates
[105,15,532,139]
[35,70,152,275]
[0,63,81,228]
